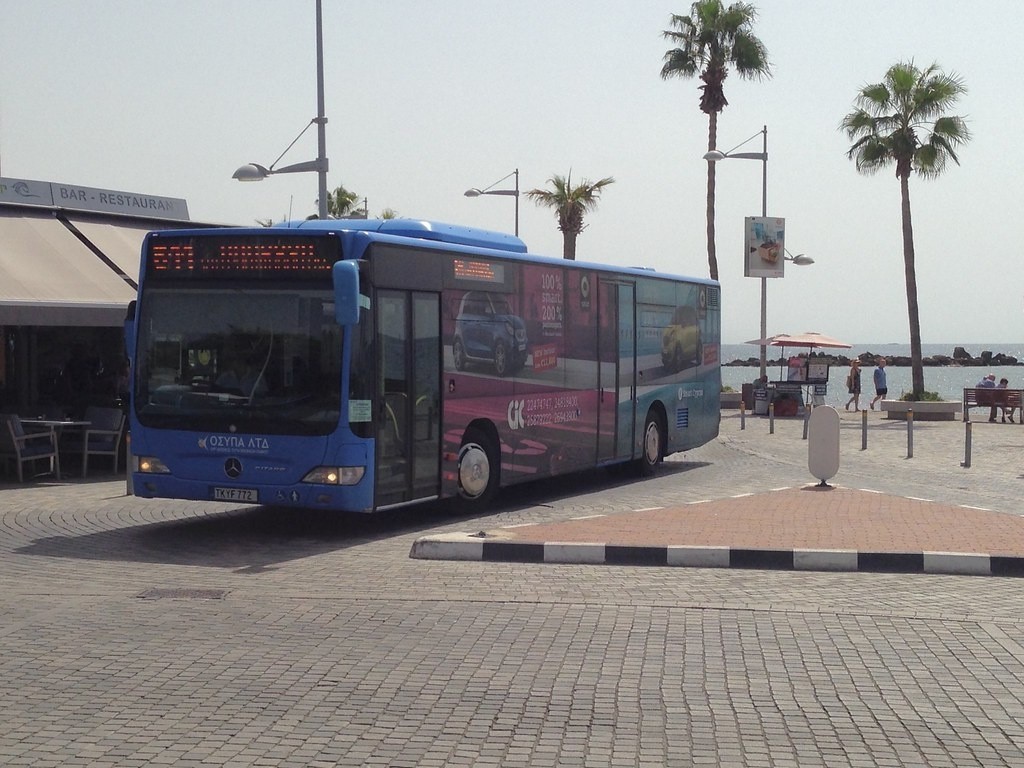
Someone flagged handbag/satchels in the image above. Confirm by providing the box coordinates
[846,375,854,388]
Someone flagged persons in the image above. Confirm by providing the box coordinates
[752,375,768,389]
[845,358,862,412]
[975,374,1012,423]
[995,378,1016,423]
[869,359,888,410]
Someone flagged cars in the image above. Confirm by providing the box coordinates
[451,290,530,377]
[660,304,702,373]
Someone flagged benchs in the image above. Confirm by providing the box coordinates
[963,387,1024,424]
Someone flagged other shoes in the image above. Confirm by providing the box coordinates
[870,402,875,410]
[1008,416,1014,422]
[1005,410,1013,414]
[846,403,849,410]
[988,418,996,422]
[855,409,861,412]
[1002,416,1006,422]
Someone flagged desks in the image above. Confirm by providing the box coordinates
[19,417,92,481]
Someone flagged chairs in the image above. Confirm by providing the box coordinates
[0,413,60,483]
[58,407,126,478]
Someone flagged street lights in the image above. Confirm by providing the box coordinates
[230,0,331,219]
[463,167,521,238]
[701,124,815,386]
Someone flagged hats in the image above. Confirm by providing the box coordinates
[850,357,861,366]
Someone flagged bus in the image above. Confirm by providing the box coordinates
[122,217,724,518]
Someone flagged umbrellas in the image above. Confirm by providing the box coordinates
[744,333,821,382]
[771,331,852,381]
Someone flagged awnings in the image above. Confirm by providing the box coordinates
[0,214,140,326]
[56,211,158,287]
